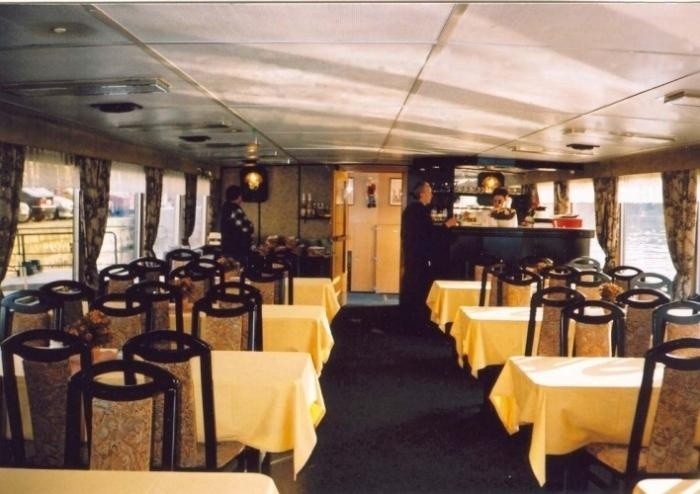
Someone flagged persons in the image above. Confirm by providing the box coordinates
[482,188,518,228]
[219,185,254,262]
[400,181,457,306]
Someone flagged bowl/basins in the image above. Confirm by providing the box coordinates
[430,215,447,225]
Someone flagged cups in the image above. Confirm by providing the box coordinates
[430,180,486,193]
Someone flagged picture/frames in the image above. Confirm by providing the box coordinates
[337,177,355,205]
[388,177,403,206]
